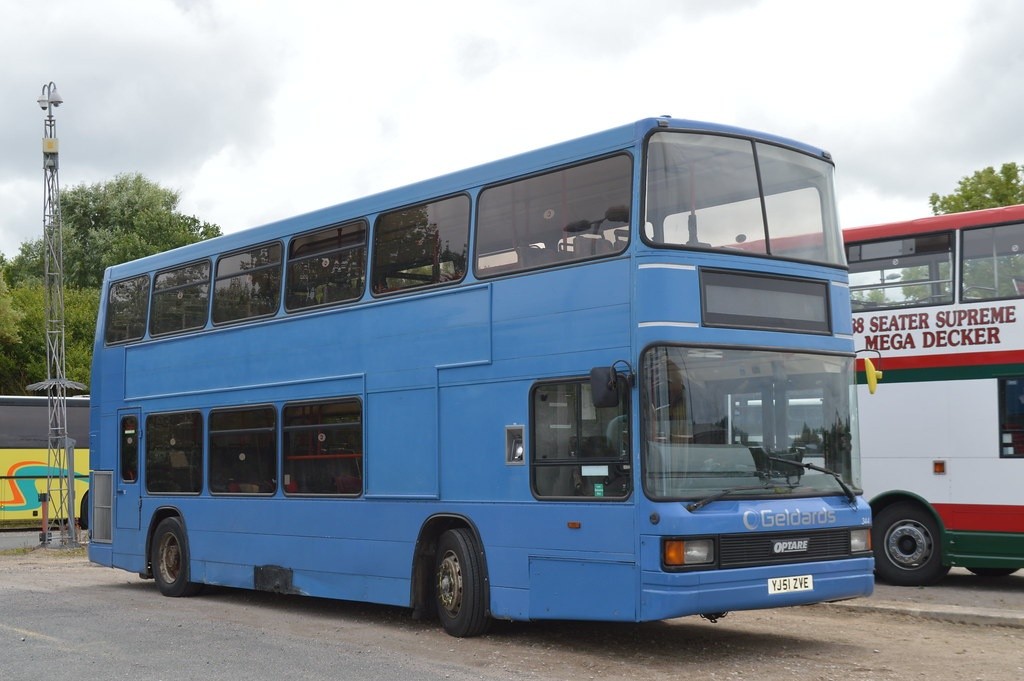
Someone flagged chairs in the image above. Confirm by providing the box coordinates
[135,232,627,334]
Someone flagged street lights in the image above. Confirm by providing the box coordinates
[35,80,78,549]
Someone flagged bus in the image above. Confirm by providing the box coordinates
[88,113,885,638]
[698,202,1024,588]
[0,394,91,531]
